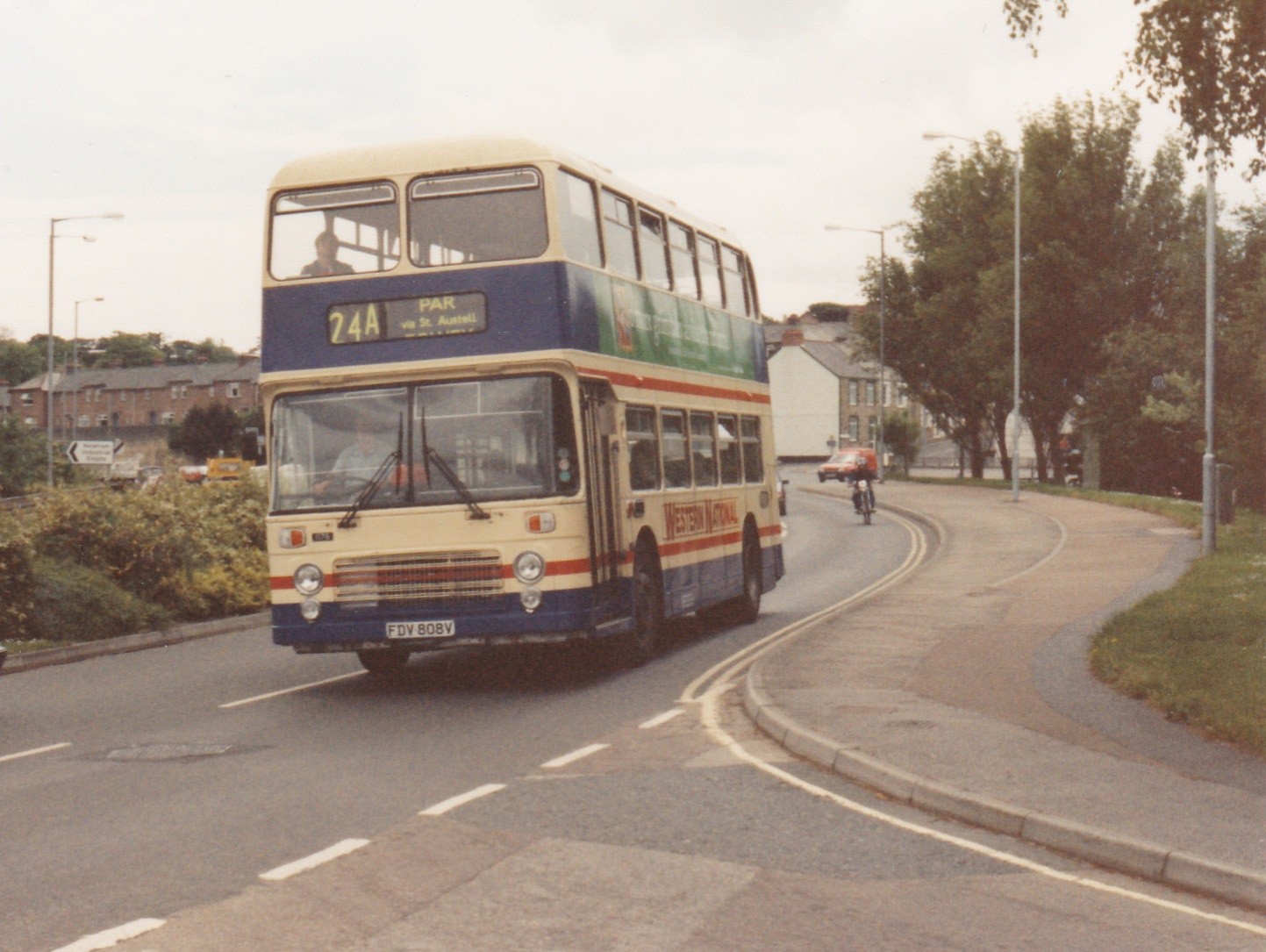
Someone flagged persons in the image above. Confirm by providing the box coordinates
[848,458,881,514]
[315,410,401,495]
[632,440,762,490]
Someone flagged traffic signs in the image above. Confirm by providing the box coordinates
[66,440,114,465]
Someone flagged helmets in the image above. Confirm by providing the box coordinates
[856,457,867,465]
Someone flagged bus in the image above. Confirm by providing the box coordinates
[258,132,786,676]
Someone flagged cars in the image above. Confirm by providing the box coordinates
[776,471,789,517]
[136,466,163,485]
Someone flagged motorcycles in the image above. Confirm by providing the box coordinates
[845,477,880,527]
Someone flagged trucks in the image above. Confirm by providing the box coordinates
[817,447,877,482]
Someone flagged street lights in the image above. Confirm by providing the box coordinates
[825,226,884,483]
[47,212,125,491]
[72,297,104,441]
[923,133,1021,503]
[61,350,107,442]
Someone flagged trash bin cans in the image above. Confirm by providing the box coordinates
[1216,463,1238,524]
